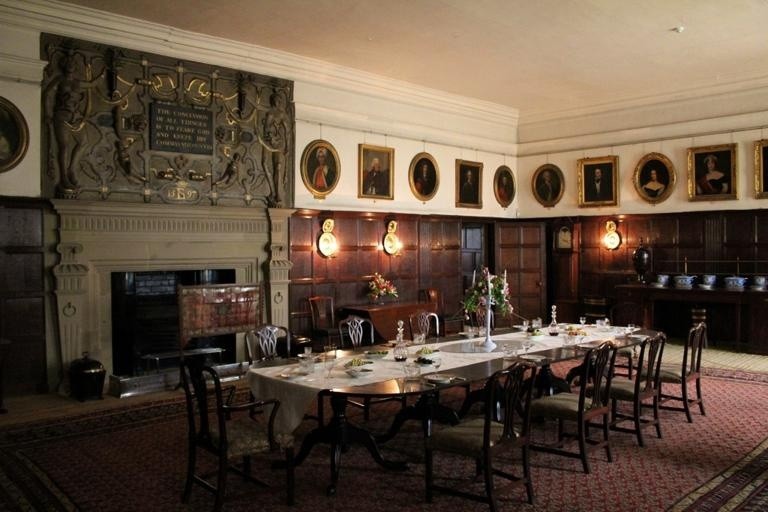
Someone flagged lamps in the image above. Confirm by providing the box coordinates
[318,218,337,256]
[603,220,620,250]
[383,221,404,255]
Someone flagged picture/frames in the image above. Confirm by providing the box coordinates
[408,151,440,201]
[686,143,740,202]
[634,152,677,205]
[752,141,768,200]
[357,143,395,200]
[531,164,564,208]
[576,156,621,209]
[0,95,30,174]
[300,140,342,200]
[455,159,483,209]
[493,165,517,208]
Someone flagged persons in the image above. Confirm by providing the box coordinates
[309,147,335,190]
[537,170,556,202]
[363,158,388,195]
[414,161,435,195]
[461,169,475,203]
[696,154,729,195]
[586,169,612,201]
[244,92,290,203]
[214,152,241,186]
[641,166,666,198]
[50,52,108,190]
[498,175,509,202]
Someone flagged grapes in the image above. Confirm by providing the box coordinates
[351,358,365,366]
[420,347,432,354]
[367,350,388,354]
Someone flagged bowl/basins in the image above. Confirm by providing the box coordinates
[656,274,670,285]
[702,274,716,285]
[753,276,767,285]
[723,274,747,286]
[672,272,697,285]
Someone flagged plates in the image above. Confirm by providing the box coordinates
[652,283,763,291]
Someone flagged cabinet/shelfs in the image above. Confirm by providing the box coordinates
[615,280,768,354]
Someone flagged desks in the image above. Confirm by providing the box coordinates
[247,323,662,500]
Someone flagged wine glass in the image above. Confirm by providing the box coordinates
[300,315,631,382]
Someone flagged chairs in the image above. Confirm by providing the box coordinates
[180,357,296,510]
[338,315,409,424]
[421,362,537,511]
[409,309,494,406]
[587,332,666,447]
[636,322,707,423]
[524,340,618,474]
[308,296,349,346]
[245,323,324,451]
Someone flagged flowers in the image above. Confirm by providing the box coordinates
[461,265,514,317]
[366,272,399,306]
